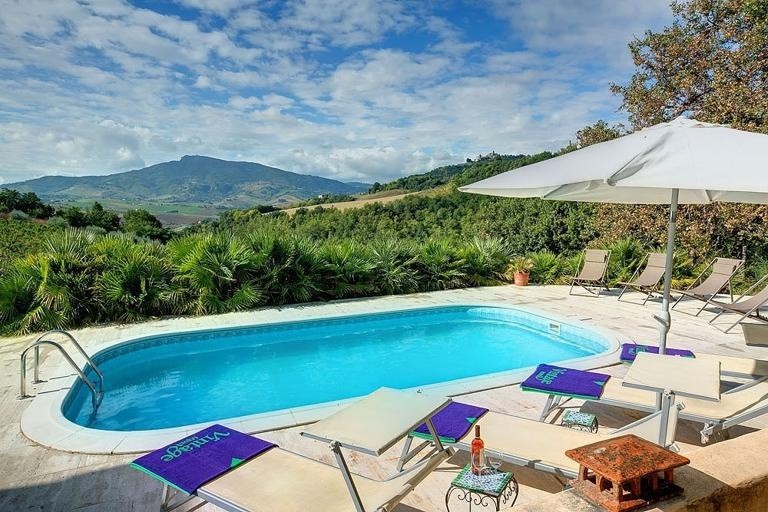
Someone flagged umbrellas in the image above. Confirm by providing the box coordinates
[457,115,768,417]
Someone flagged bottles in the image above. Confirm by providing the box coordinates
[470,424,486,475]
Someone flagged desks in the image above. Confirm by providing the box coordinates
[445,462,518,512]
[559,410,599,433]
[299,388,451,512]
[621,352,720,410]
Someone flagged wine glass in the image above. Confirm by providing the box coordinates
[470,452,503,486]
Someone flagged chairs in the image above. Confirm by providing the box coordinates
[617,252,677,305]
[620,343,768,380]
[708,274,768,333]
[568,249,612,298]
[395,392,685,488]
[521,364,768,443]
[126,424,456,512]
[672,256,746,316]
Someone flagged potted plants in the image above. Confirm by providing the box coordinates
[502,254,536,286]
